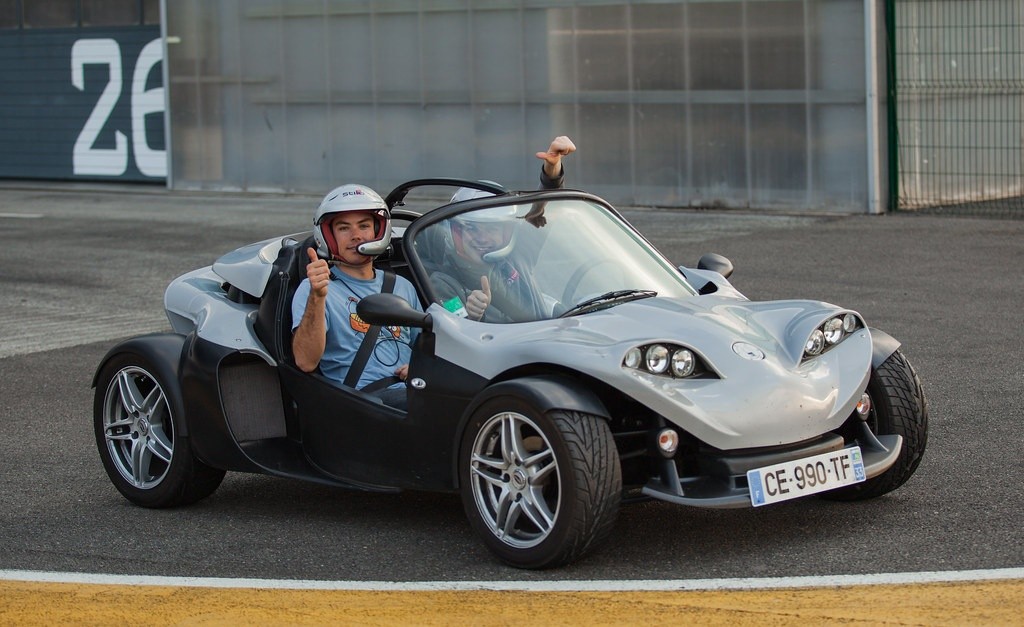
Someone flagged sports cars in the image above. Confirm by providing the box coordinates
[91,179,930,569]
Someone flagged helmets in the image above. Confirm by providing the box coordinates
[314,184,392,260]
[444,179,519,262]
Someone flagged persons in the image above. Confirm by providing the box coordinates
[291,184,425,410]
[431,136,576,324]
